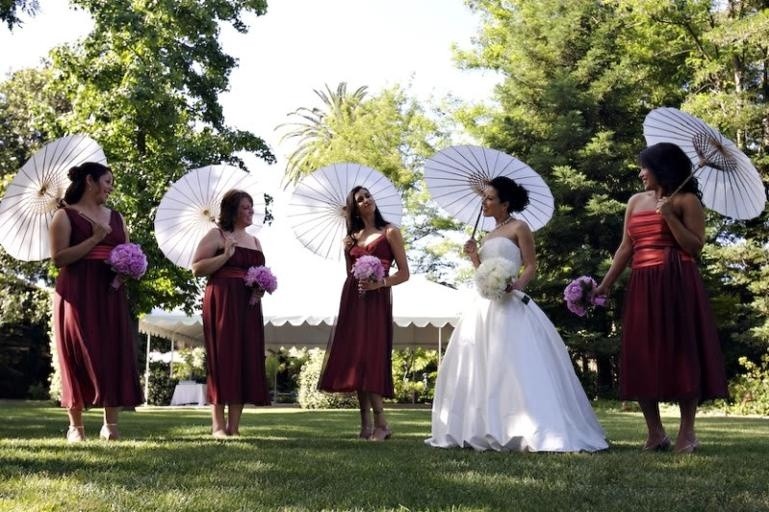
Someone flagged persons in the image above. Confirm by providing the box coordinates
[595,145,728,452]
[192,190,270,440]
[47,162,144,440]
[318,188,409,443]
[427,179,608,452]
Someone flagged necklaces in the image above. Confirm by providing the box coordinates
[489,216,514,230]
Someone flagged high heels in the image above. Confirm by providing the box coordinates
[670,436,700,453]
[67,429,86,445]
[641,433,672,453]
[98,429,120,445]
[357,427,372,440]
[367,422,392,442]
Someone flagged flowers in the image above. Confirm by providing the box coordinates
[244,265,277,305]
[104,244,148,291]
[350,255,384,297]
[563,275,609,317]
[473,257,530,304]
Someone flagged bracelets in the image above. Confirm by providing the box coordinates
[382,277,386,288]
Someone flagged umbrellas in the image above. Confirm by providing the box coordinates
[155,166,266,270]
[291,164,403,262]
[0,134,109,262]
[641,107,767,221]
[426,147,555,251]
